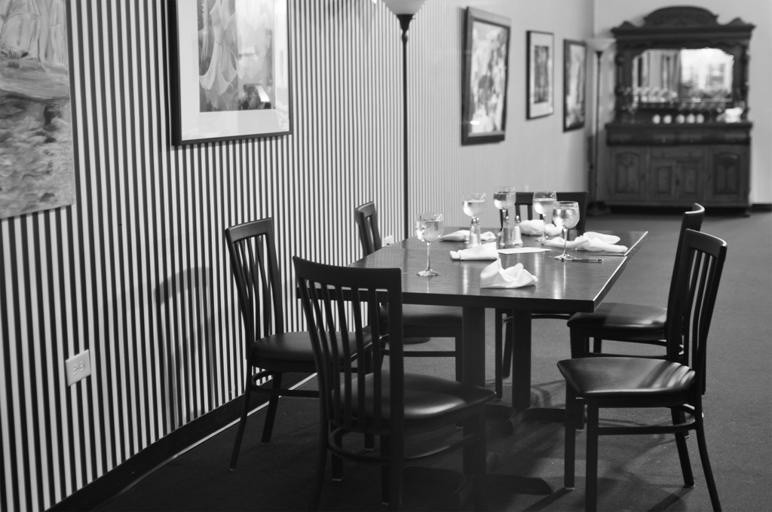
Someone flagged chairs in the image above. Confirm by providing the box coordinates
[224,218,389,484]
[293,254,498,511]
[558,228,727,511]
[354,202,464,382]
[567,203,705,435]
[500,191,602,379]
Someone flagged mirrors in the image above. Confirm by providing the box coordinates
[630,45,737,109]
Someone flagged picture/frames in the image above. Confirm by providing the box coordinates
[562,38,587,133]
[462,5,511,146]
[526,30,555,120]
[168,0,295,146]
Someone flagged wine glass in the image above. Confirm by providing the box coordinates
[532,190,558,242]
[461,192,489,247]
[552,200,580,260]
[415,214,444,277]
[493,186,517,237]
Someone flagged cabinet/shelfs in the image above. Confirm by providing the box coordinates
[604,6,756,209]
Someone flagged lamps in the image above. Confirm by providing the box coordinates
[586,37,617,216]
[382,0,427,243]
